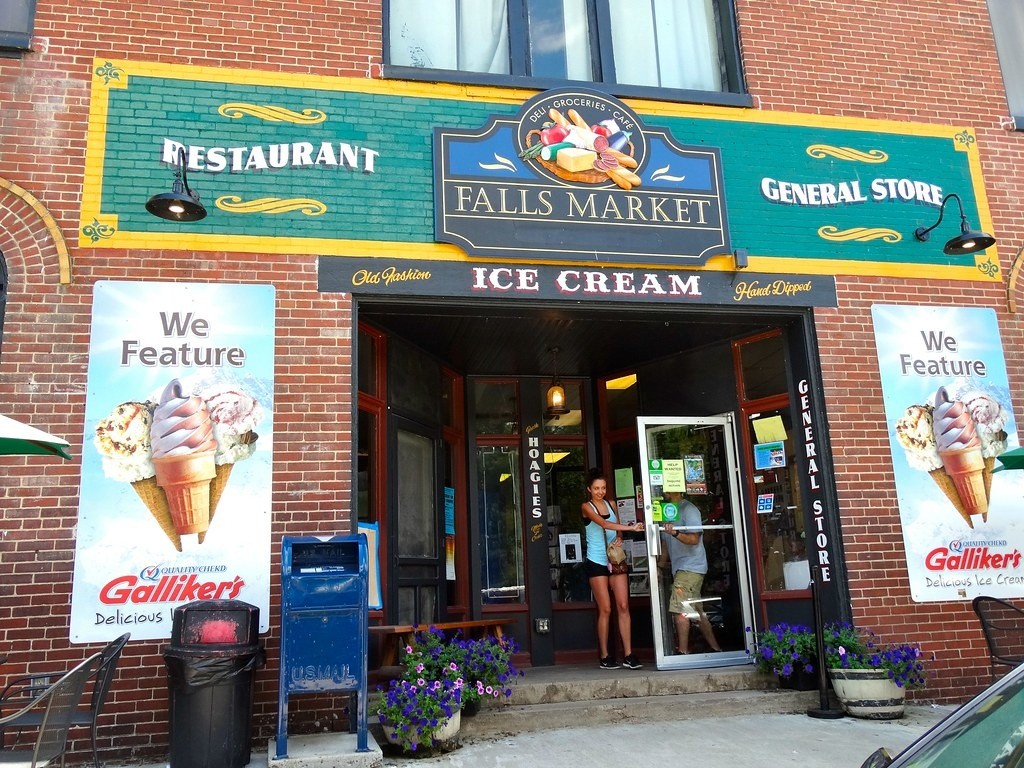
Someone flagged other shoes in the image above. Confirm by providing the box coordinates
[704,648,723,653]
[673,651,693,655]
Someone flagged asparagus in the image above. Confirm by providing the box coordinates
[518,142,545,162]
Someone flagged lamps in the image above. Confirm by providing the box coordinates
[547,347,570,416]
[915,194,996,256]
[146,146,209,221]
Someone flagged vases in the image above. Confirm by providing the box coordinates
[378,708,461,759]
[790,656,821,691]
[461,697,482,716]
[828,668,907,720]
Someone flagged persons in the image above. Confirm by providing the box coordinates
[657,481,723,655]
[581,468,645,670]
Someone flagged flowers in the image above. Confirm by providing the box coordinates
[368,621,525,753]
[744,620,936,688]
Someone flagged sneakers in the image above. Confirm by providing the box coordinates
[600,655,622,669]
[623,652,643,668]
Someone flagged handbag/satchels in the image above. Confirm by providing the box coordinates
[611,560,628,574]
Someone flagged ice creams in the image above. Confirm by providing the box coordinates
[93,378,265,552]
[895,386,1008,529]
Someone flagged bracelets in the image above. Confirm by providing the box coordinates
[657,566,664,572]
[672,530,680,537]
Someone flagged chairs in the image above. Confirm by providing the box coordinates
[972,595,1024,681]
[0,632,131,767]
[0,652,102,768]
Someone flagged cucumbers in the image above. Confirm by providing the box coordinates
[540,142,576,161]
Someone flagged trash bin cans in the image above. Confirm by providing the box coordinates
[161,599,267,768]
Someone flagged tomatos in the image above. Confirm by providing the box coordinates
[590,122,613,138]
[540,121,570,146]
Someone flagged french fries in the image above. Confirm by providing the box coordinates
[549,107,641,191]
